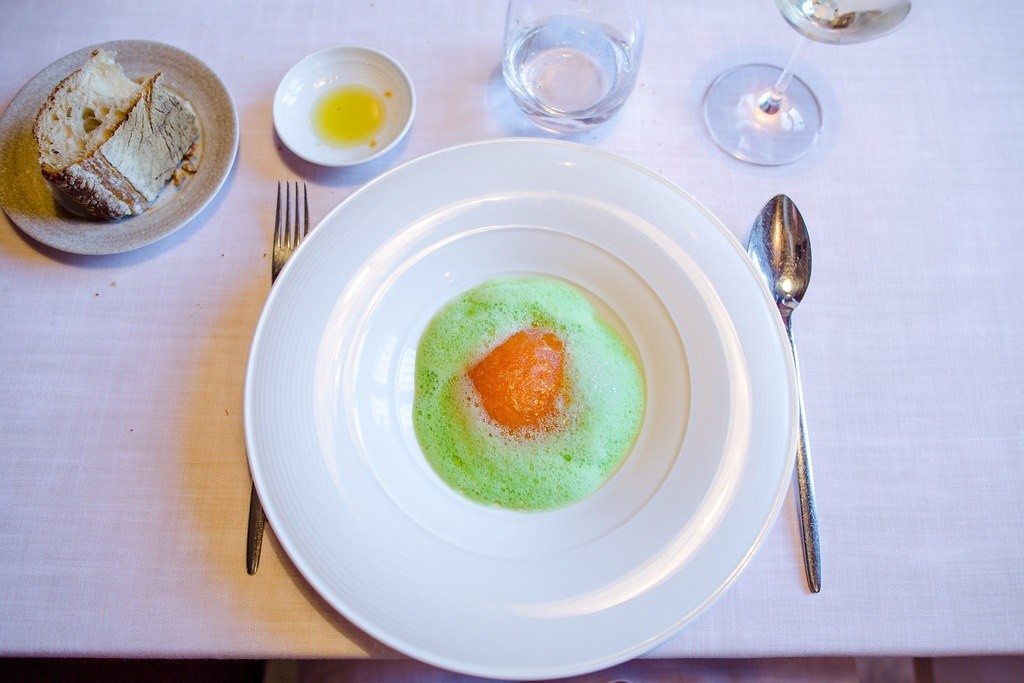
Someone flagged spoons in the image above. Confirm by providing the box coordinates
[747,195,820,594]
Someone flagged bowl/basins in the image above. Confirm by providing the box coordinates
[272,46,416,167]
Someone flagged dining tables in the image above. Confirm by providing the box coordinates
[1,2,1024,683]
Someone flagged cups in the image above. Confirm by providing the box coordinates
[502,0,648,135]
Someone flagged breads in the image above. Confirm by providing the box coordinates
[33,49,200,219]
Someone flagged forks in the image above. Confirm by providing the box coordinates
[247,182,310,576]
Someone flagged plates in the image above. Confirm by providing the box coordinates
[0,39,239,256]
[243,137,799,680]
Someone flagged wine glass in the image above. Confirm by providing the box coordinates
[704,1,917,167]
